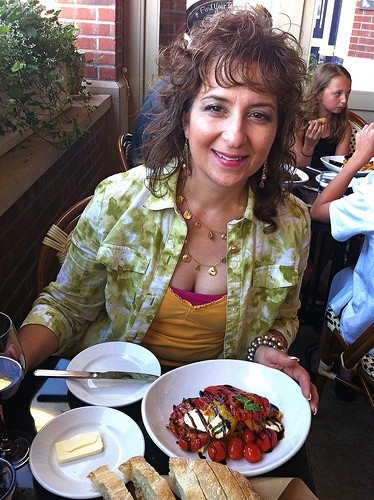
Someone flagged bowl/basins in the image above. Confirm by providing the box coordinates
[278,162,309,194]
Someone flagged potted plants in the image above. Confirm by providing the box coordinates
[0,0,99,165]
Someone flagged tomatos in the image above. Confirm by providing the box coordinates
[176,428,277,464]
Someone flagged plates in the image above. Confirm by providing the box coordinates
[315,172,359,189]
[140,358,313,477]
[319,153,372,175]
[65,340,161,407]
[30,406,145,500]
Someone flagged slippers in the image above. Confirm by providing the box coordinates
[334,346,357,400]
[302,338,329,386]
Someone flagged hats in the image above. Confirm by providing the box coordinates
[187,1,234,34]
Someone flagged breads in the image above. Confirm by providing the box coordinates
[85,455,267,500]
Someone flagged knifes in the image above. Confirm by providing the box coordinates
[34,368,158,383]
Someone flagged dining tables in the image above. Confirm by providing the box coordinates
[279,160,367,333]
[0,354,317,500]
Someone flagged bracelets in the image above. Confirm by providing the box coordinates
[247,334,288,365]
[301,149,313,158]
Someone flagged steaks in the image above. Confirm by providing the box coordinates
[172,384,279,438]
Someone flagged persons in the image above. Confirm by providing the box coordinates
[131,73,175,168]
[307,120,374,373]
[1,1,322,416]
[285,61,352,308]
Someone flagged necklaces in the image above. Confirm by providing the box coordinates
[176,191,244,276]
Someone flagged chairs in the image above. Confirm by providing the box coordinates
[34,196,93,303]
[118,131,136,173]
[347,110,368,159]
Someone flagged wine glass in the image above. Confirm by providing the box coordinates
[0,310,36,471]
